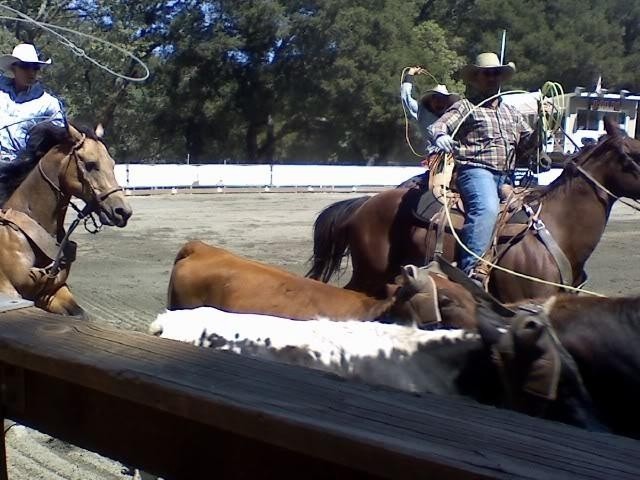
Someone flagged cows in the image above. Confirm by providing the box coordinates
[476,294,640,440]
[131,305,508,480]
[166,240,478,329]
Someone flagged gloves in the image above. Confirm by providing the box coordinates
[433,132,459,152]
[537,98,556,118]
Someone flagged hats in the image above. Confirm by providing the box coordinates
[464,53,516,80]
[418,84,463,112]
[0,43,52,72]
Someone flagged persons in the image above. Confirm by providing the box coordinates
[0,43,65,211]
[400,63,462,147]
[426,53,548,293]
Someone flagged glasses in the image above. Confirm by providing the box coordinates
[481,68,502,77]
[12,62,41,70]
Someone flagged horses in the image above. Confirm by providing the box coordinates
[0,117,133,316]
[302,116,640,299]
